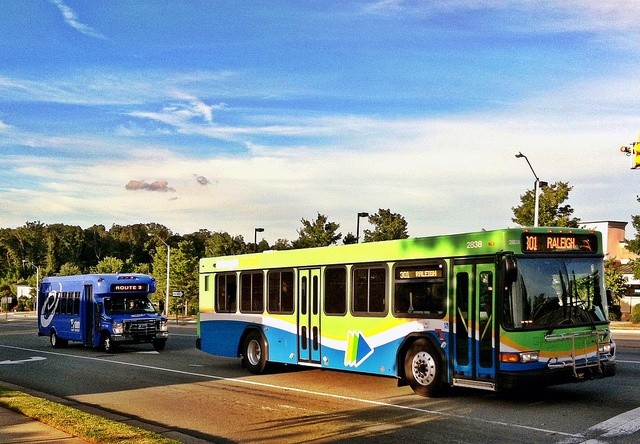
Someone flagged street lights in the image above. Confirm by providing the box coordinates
[148,233,170,317]
[254,228,264,252]
[515,151,547,227]
[22,260,39,316]
[357,212,369,242]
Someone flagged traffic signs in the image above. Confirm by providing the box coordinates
[172,290,185,298]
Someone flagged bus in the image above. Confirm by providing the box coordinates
[38,273,168,353]
[196,228,616,397]
